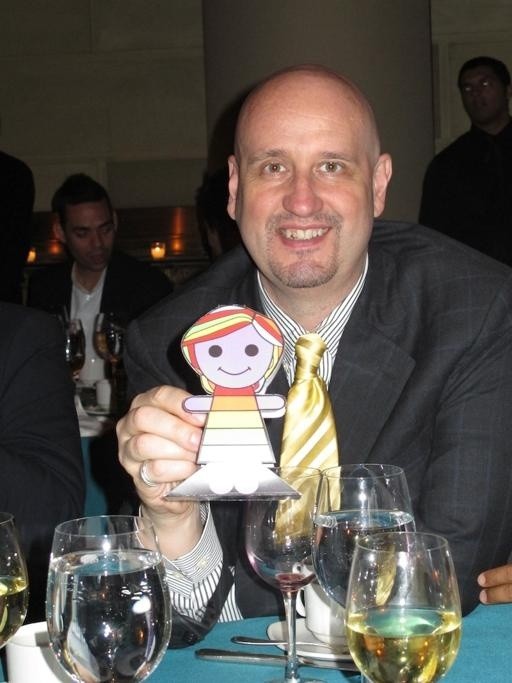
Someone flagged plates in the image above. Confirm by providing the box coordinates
[84,404,113,415]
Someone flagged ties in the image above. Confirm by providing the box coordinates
[273,335,342,540]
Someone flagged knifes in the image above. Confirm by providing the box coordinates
[194,648,361,675]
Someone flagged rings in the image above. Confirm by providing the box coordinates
[139,460,157,487]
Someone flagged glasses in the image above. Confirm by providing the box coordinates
[462,78,492,94]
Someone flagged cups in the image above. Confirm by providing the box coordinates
[345,530,461,682]
[96,378,111,411]
[0,513,173,683]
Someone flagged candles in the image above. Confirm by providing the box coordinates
[151,247,166,260]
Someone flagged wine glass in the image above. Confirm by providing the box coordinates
[95,311,127,387]
[246,464,418,682]
[64,318,86,378]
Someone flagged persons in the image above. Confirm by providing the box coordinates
[196,172,240,261]
[476,563,511,604]
[418,56,512,268]
[95,63,511,650]
[18,174,177,535]
[0,298,90,630]
